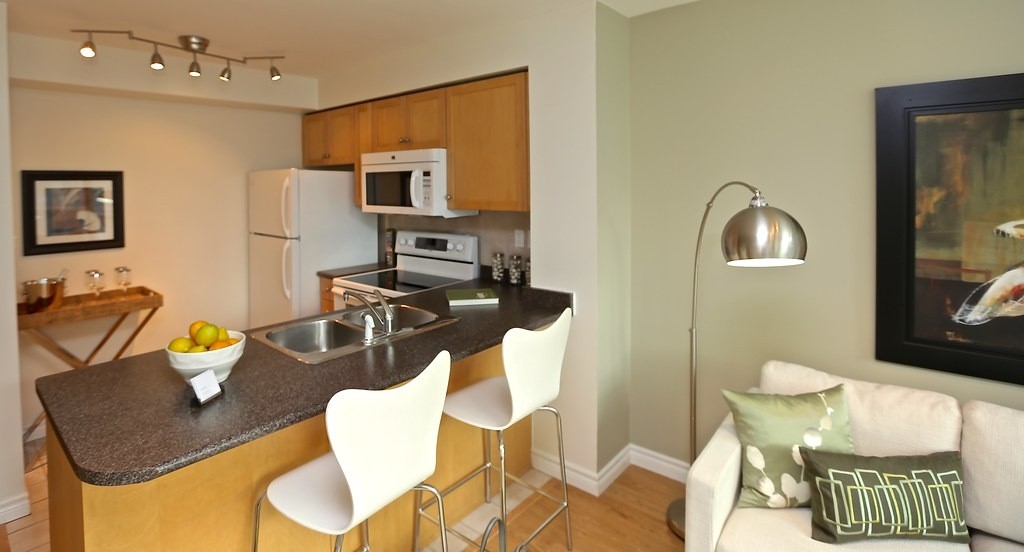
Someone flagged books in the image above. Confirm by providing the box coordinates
[445,288,499,306]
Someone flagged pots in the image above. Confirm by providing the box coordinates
[22,278,67,314]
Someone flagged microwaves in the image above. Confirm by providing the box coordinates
[361,148,479,219]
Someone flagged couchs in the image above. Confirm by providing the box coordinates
[684,360,1024,552]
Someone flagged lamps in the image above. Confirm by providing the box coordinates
[80,31,96,58]
[189,52,201,77]
[149,43,165,71]
[219,60,232,82]
[269,58,281,81]
[667,181,809,541]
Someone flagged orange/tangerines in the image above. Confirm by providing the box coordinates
[190,321,241,350]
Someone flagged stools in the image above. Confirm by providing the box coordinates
[252,350,452,552]
[413,306,573,551]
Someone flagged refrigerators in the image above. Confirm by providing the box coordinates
[248,168,378,329]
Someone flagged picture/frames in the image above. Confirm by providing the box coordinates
[874,72,1024,386]
[21,170,125,255]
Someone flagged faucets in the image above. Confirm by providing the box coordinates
[343,289,395,333]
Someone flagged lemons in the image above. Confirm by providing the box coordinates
[168,324,229,353]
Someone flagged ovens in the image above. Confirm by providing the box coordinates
[331,287,391,310]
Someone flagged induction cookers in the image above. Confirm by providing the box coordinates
[333,231,479,298]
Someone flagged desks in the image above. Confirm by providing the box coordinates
[17,286,163,472]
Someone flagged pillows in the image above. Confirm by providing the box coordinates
[720,382,854,509]
[798,446,971,544]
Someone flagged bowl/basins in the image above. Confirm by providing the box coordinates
[165,330,246,387]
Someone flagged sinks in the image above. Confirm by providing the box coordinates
[342,304,440,332]
[265,318,366,354]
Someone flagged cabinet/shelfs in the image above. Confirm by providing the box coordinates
[320,276,334,314]
[301,70,531,210]
